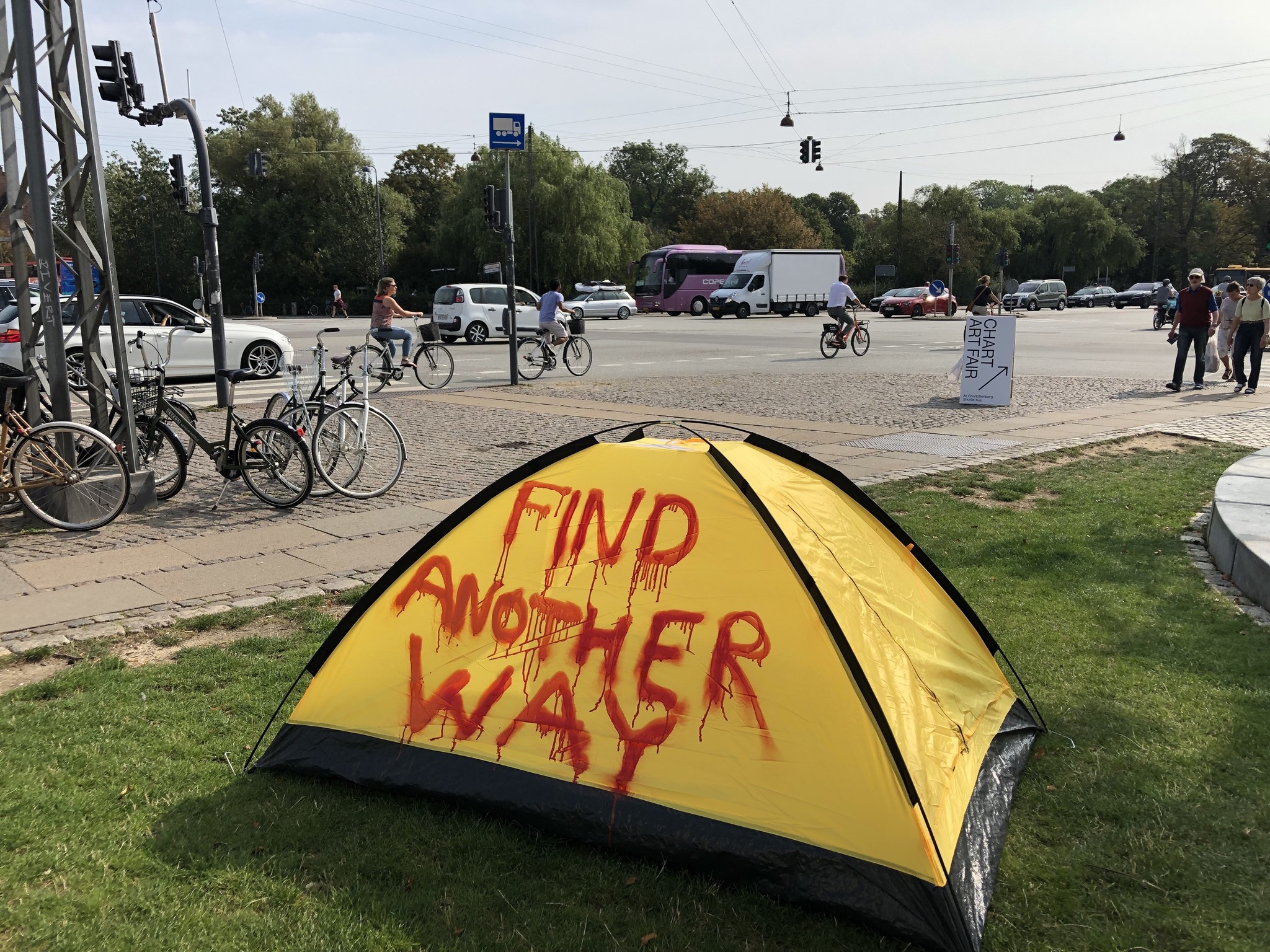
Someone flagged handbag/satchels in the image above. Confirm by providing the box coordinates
[966,301,974,314]
[1204,335,1220,373]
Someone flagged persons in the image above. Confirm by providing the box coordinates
[537,278,575,370]
[668,272,674,283]
[971,275,1003,316]
[827,274,866,345]
[1216,280,1246,382]
[1156,279,1175,317]
[924,282,930,286]
[1166,268,1219,391]
[516,302,525,305]
[1227,277,1270,394]
[752,276,761,287]
[329,285,348,319]
[1120,283,1125,292]
[1094,283,1102,287]
[370,277,423,386]
[147,309,172,326]
[1218,275,1231,308]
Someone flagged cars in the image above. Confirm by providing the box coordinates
[868,285,960,316]
[1003,278,1248,330]
[0,280,48,312]
[561,290,638,320]
[431,284,572,346]
[0,295,295,394]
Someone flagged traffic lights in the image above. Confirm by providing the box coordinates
[947,243,961,266]
[484,184,509,230]
[800,140,822,163]
[245,151,268,178]
[192,256,206,275]
[92,39,145,102]
[253,253,265,273]
[169,155,190,207]
[996,253,1009,268]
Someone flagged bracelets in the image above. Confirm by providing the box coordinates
[1212,324,1216,327]
[1263,333,1268,336]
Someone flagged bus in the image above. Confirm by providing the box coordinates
[0,256,100,297]
[628,244,755,316]
[1212,266,1270,300]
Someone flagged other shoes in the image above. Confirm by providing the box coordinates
[344,315,348,320]
[329,315,334,319]
[1165,313,1170,317]
[836,336,847,345]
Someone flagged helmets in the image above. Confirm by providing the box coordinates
[1163,279,1170,284]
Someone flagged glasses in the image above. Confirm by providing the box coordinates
[1189,276,1202,280]
[333,287,336,288]
[387,284,396,287]
[847,281,848,282]
[1246,284,1254,287]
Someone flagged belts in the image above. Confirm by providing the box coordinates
[1240,320,1264,324]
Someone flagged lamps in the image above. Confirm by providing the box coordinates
[471,135,482,161]
[1028,175,1034,192]
[780,92,794,127]
[1231,189,1238,203]
[816,158,824,171]
[1114,114,1125,140]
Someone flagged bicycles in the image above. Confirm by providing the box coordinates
[244,301,260,316]
[0,317,593,532]
[820,304,870,358]
[300,296,345,317]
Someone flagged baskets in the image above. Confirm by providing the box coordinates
[108,367,166,413]
[418,322,442,342]
[278,349,319,394]
[567,319,585,335]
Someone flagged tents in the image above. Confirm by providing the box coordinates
[244,419,1050,952]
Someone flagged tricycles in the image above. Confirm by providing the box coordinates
[1153,295,1180,332]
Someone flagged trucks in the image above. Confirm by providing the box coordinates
[707,248,842,318]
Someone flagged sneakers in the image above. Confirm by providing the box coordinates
[544,364,557,370]
[545,342,556,357]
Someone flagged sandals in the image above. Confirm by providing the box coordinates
[1222,368,1232,380]
[1166,382,1181,391]
[1245,387,1256,394]
[1225,376,1237,382]
[1194,383,1204,389]
[377,373,391,386]
[1234,382,1246,392]
[400,359,418,368]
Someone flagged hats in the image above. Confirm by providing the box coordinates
[1189,268,1204,276]
[1224,276,1231,282]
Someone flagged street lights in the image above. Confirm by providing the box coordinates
[362,165,384,281]
[138,194,161,296]
